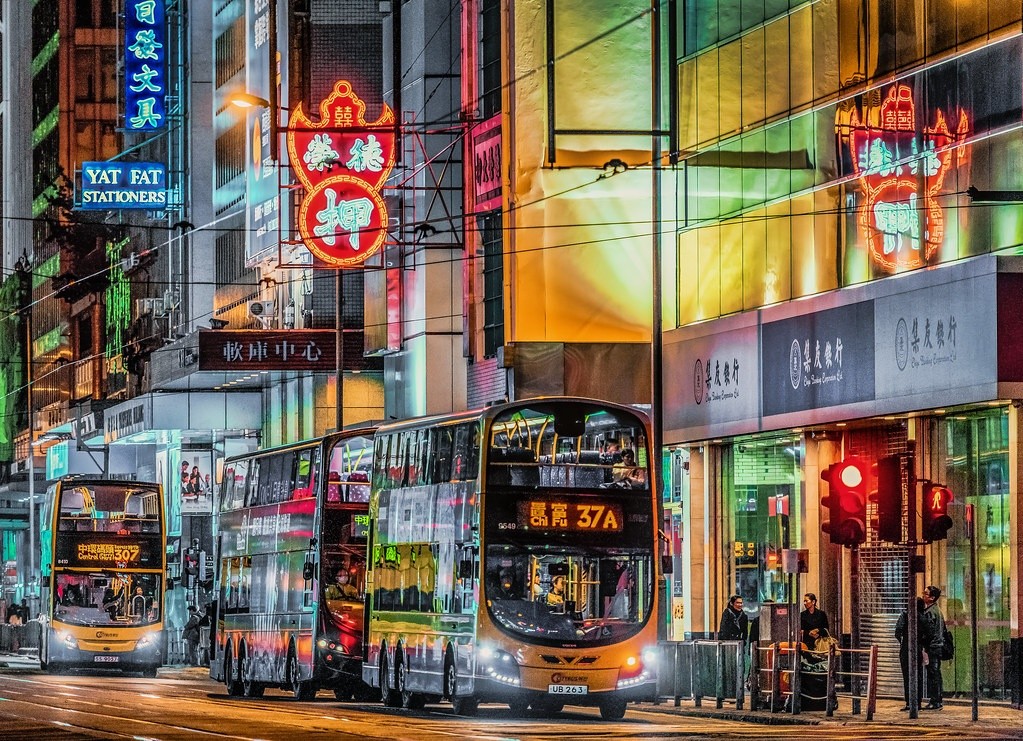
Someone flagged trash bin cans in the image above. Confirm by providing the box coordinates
[765,642,807,696]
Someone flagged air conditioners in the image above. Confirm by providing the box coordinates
[246,300,275,317]
[137,290,180,316]
[122,252,140,272]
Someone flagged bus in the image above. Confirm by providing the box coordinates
[362,396,675,722]
[39,478,174,677]
[222,420,377,701]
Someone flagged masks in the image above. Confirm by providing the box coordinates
[336,575,348,584]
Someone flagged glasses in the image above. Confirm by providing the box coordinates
[922,590,930,596]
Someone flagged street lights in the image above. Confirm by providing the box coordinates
[28,434,59,618]
[232,94,344,433]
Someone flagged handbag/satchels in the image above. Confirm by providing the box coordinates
[942,628,954,661]
[814,628,841,656]
[922,648,929,666]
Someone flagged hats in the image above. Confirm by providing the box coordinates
[187,605,196,611]
[551,575,564,583]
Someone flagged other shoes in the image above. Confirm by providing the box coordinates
[900,706,910,712]
[924,703,943,711]
[918,706,923,712]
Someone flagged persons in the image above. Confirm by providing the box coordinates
[718,595,748,666]
[800,593,839,711]
[895,597,933,711]
[612,449,644,481]
[102,584,124,621]
[181,460,211,504]
[611,589,628,619]
[130,586,147,617]
[746,599,774,689]
[599,438,623,462]
[528,571,543,594]
[182,606,203,666]
[0,598,30,653]
[65,588,82,607]
[922,585,946,711]
[325,567,361,601]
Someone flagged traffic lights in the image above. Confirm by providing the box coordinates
[821,460,867,549]
[200,551,213,586]
[923,484,953,543]
[185,547,200,576]
[869,453,903,545]
